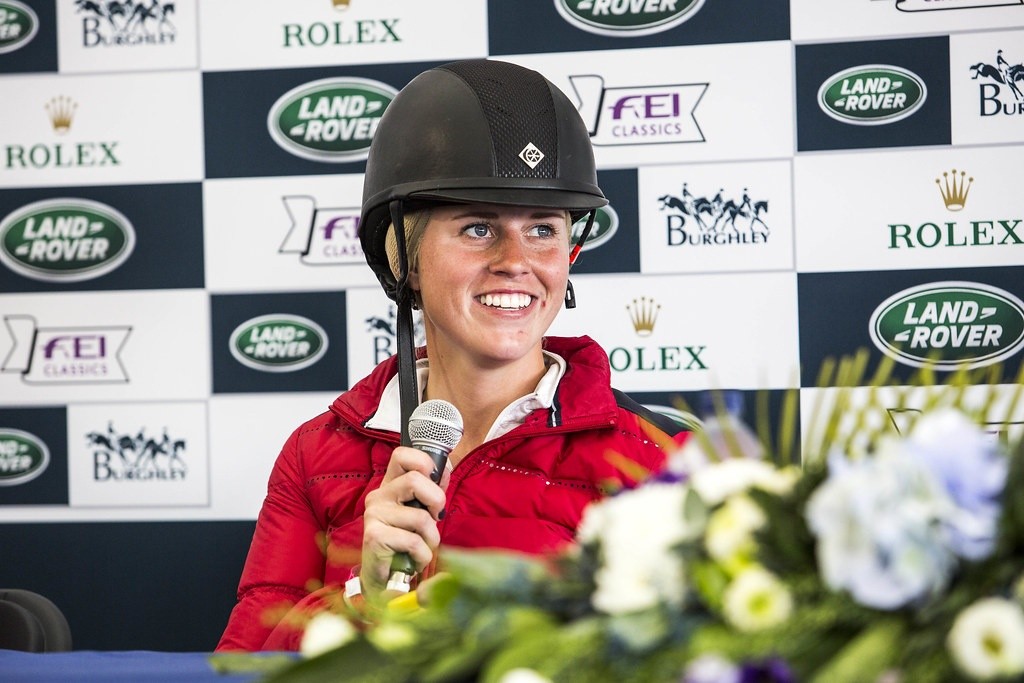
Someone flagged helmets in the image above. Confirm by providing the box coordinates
[358,58,609,304]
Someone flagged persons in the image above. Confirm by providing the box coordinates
[214,60,704,652]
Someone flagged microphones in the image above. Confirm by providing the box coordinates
[388,400,463,590]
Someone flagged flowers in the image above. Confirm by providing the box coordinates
[208,342,1024,683]
